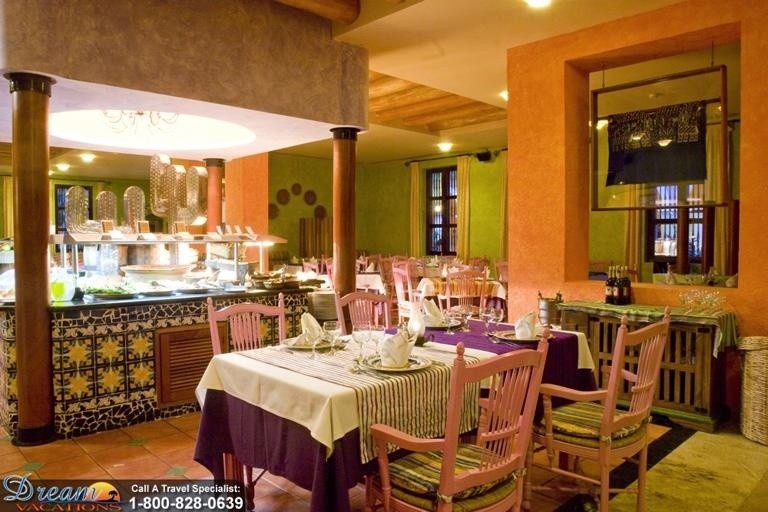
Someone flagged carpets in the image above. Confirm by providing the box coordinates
[552,426,768,512]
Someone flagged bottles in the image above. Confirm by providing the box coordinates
[604,265,632,305]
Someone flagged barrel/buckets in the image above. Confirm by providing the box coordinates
[537,296,564,329]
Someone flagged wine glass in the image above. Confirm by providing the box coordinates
[322,320,341,359]
[369,325,387,362]
[675,273,729,314]
[302,326,323,361]
[445,304,500,346]
[351,322,371,363]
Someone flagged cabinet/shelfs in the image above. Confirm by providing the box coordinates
[557,300,734,433]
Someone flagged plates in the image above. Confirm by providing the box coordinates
[492,329,554,342]
[223,287,248,293]
[176,288,210,295]
[92,292,139,299]
[281,337,343,350]
[421,318,460,329]
[364,354,432,373]
[139,289,176,298]
